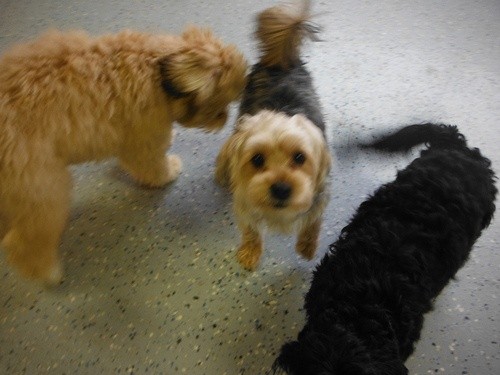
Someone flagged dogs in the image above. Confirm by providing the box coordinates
[272,123,500,375]
[214,0,332,271]
[0,26,250,287]
